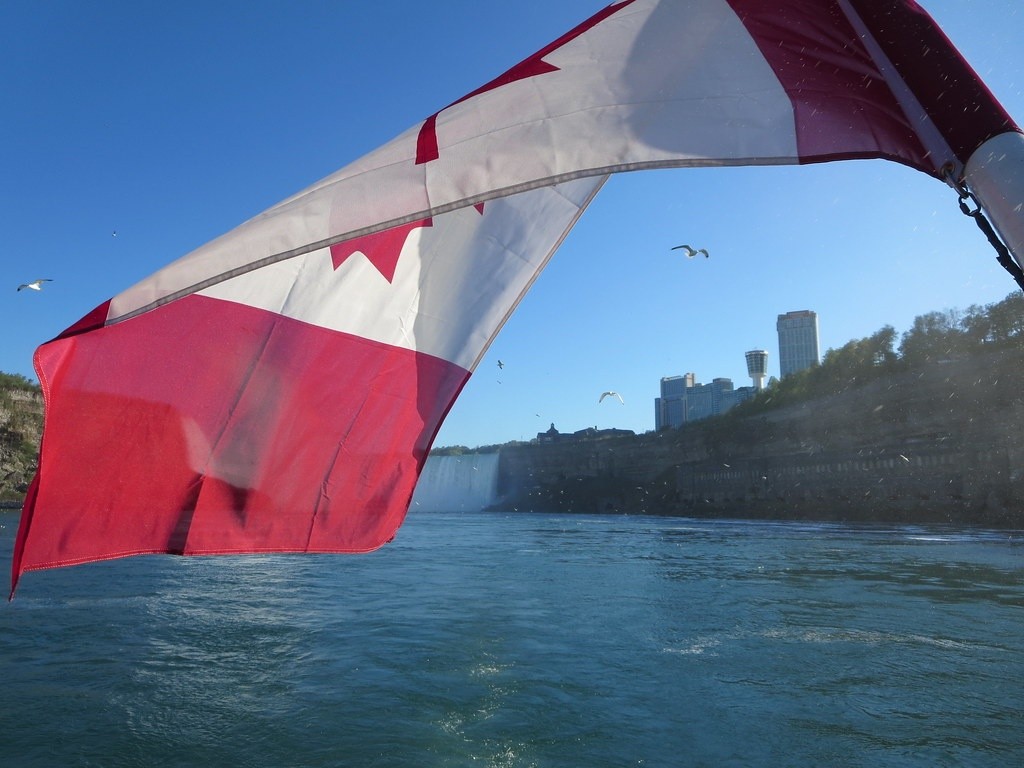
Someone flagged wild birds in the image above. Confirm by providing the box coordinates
[494,357,507,389]
[111,229,119,239]
[597,389,628,408]
[16,277,55,293]
[669,243,712,262]
[533,411,541,421]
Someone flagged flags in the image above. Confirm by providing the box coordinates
[10,0,959,599]
